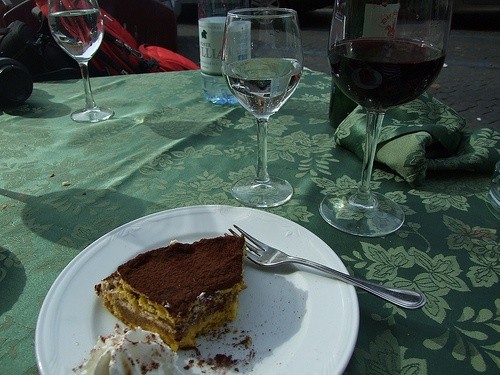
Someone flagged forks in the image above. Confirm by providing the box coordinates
[224,222,427,310]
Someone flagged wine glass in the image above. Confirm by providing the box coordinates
[320,0,454,237]
[221,7,303,209]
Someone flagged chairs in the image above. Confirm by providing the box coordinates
[95,0,177,53]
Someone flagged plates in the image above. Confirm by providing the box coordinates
[34,204,360,374]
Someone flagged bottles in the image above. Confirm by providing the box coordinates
[326,0,403,129]
[196,0,253,107]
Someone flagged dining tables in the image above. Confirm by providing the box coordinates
[0,59,500,375]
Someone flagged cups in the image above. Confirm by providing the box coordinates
[46,0,114,123]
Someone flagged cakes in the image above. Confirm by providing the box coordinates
[95,235,248,350]
